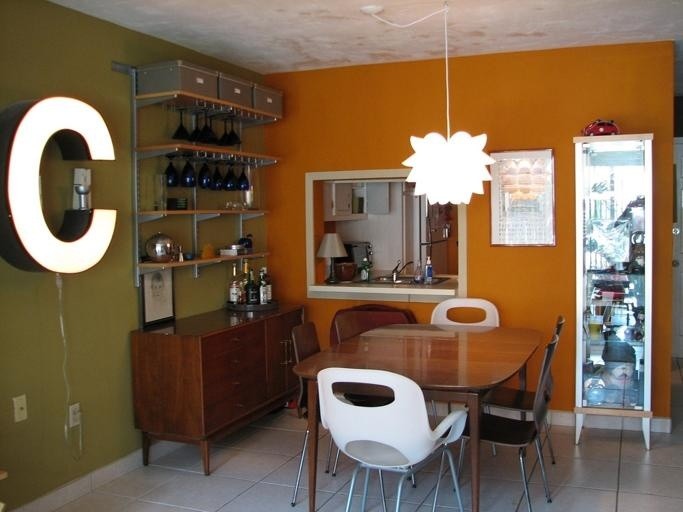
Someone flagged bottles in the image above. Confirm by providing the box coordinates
[425,256,432,282]
[227,261,272,306]
[414,261,424,282]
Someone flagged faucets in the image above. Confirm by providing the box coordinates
[389,259,414,284]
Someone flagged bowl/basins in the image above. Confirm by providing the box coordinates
[334,263,357,280]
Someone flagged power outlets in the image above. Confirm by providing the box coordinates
[68,400,81,430]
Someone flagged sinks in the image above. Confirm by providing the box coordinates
[369,275,452,287]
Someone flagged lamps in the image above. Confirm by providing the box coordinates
[0,94,119,276]
[400,1,495,207]
[314,231,349,285]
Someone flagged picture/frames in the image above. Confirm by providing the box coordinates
[139,267,176,333]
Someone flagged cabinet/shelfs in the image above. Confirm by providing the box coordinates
[129,301,304,477]
[319,182,388,222]
[571,133,656,450]
[128,66,282,287]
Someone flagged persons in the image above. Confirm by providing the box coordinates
[145,273,172,321]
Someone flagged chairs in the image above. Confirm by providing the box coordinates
[430,297,499,459]
[316,367,469,512]
[292,323,416,512]
[334,307,438,426]
[450,314,567,509]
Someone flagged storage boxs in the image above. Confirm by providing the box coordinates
[220,73,254,108]
[255,82,286,114]
[136,61,220,101]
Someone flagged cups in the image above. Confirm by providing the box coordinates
[587,323,602,340]
[222,200,248,211]
[243,185,253,208]
[153,174,167,210]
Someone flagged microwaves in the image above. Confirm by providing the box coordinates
[336,241,374,271]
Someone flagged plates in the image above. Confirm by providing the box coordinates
[155,197,188,210]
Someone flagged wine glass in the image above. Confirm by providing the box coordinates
[171,106,240,146]
[163,154,248,192]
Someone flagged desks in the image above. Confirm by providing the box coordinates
[294,325,534,508]
